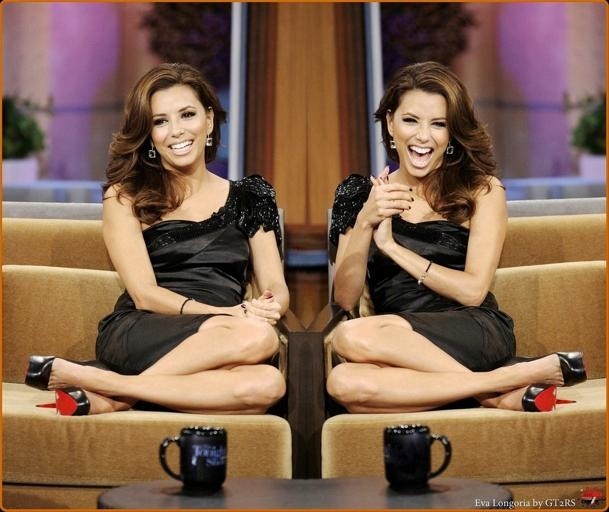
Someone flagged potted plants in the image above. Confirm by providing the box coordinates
[562,91,605,178]
[2,94,56,182]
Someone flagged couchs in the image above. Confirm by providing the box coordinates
[2,200,292,510]
[322,197,607,510]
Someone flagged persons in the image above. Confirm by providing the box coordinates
[24,63,291,418]
[325,61,587,414]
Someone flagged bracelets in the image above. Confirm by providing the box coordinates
[415,260,432,285]
[179,297,194,317]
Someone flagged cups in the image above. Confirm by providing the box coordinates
[382,424,452,492]
[159,426,228,494]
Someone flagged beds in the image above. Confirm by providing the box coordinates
[97,473,512,510]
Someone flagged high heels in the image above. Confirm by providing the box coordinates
[521,382,576,412]
[23,355,108,391]
[504,351,588,388]
[35,387,91,416]
